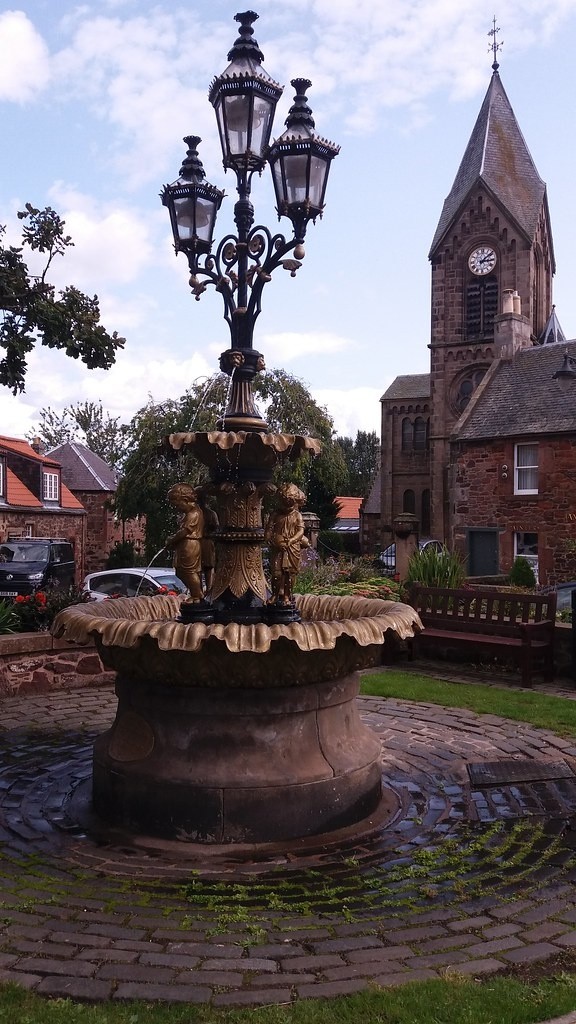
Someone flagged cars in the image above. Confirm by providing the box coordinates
[378,538,450,582]
[527,582,576,622]
[78,567,189,605]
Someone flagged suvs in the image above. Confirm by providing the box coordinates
[0,536,76,602]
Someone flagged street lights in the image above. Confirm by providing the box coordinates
[155,12,344,614]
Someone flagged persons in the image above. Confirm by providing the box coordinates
[194,486,219,591]
[265,483,307,607]
[163,482,205,604]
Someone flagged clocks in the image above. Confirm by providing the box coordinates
[468,247,496,275]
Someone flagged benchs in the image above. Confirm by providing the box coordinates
[393,584,557,688]
